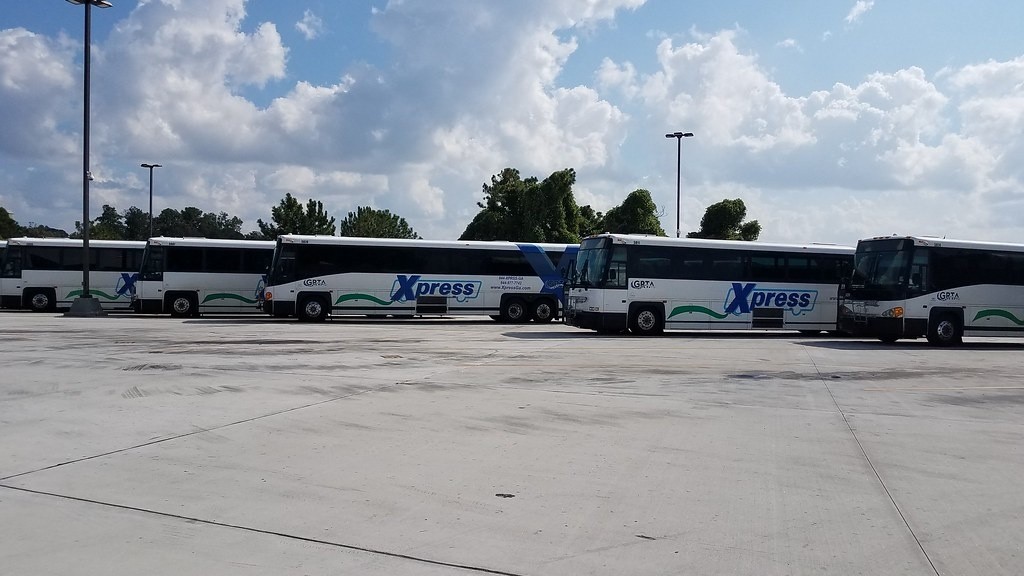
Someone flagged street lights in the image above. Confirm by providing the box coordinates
[70,2,111,317]
[141,163,163,236]
[665,131,694,236]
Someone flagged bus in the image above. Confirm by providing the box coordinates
[134,235,278,318]
[0,235,165,312]
[561,233,854,337]
[843,236,1024,346]
[259,234,576,324]
